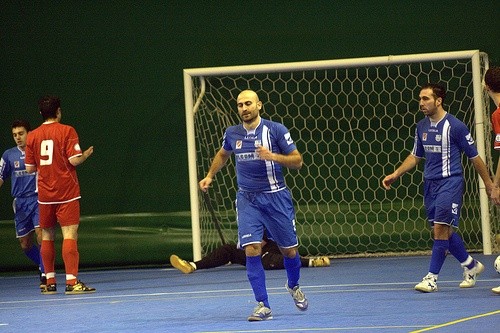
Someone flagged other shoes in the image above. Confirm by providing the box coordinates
[492,286,500,293]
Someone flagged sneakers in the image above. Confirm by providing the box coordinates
[285,280,308,311]
[248,302,273,321]
[310,256,330,267]
[415,272,438,292]
[459,260,484,287]
[41,283,57,295]
[40,276,46,288]
[170,255,196,273]
[65,280,96,295]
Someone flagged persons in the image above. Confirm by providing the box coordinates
[198,89,308,321]
[484,66,500,293]
[24,95,97,295]
[170,230,331,274]
[0,119,47,284]
[382,82,496,293]
